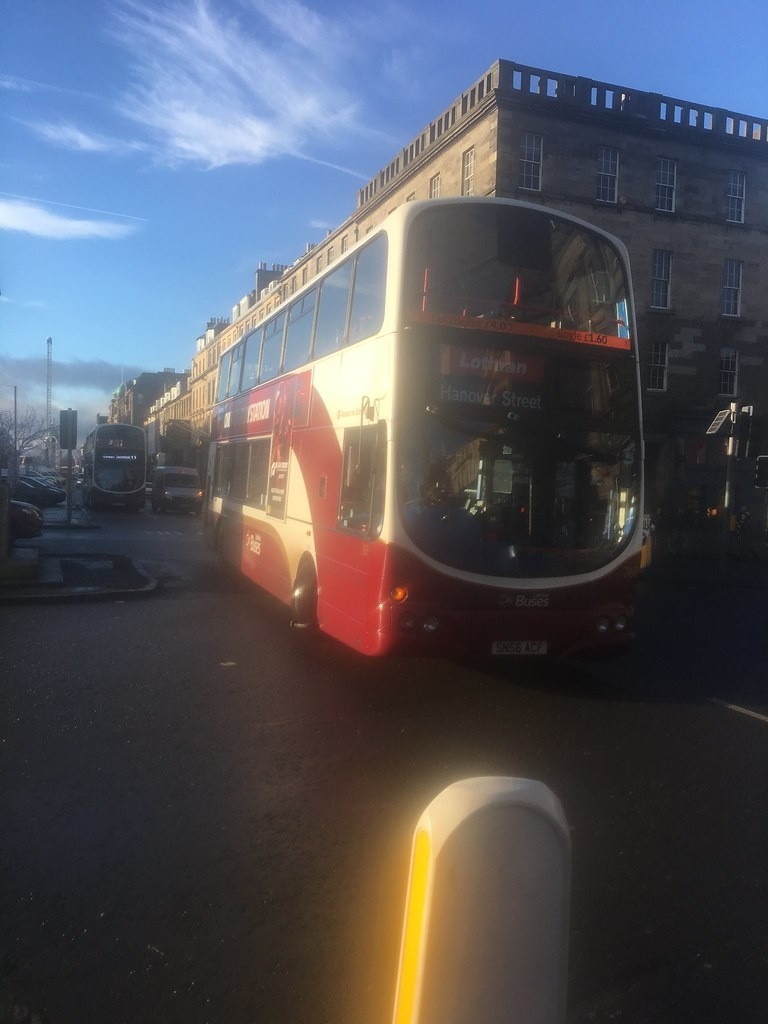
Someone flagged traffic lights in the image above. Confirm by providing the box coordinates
[518,492,528,515]
[753,456,768,489]
[737,405,766,461]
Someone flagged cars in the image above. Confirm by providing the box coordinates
[1,465,70,548]
[146,482,153,500]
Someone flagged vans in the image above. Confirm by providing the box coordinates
[152,465,203,516]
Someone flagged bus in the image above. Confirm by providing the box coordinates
[82,423,146,512]
[201,196,647,671]
